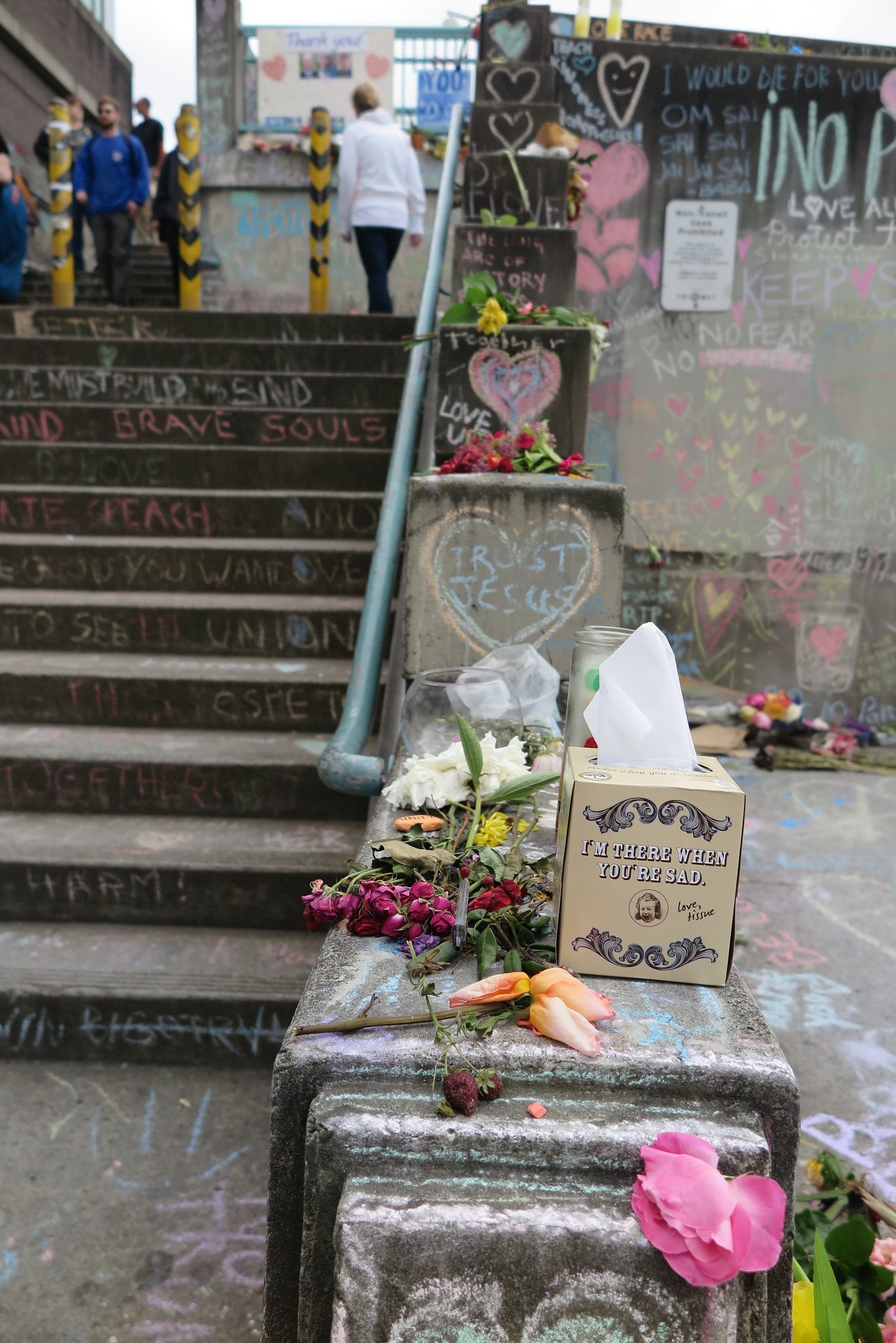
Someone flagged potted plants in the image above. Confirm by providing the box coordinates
[407,116,435,150]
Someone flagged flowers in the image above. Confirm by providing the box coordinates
[733,691,858,757]
[413,419,605,481]
[297,710,615,1115]
[400,271,608,352]
[629,1129,896,1343]
[731,31,787,51]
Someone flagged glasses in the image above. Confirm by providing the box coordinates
[98,109,117,114]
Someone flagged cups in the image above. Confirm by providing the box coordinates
[561,625,644,762]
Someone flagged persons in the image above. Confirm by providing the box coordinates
[131,98,180,309]
[0,133,37,304]
[337,83,427,314]
[33,94,99,272]
[73,95,151,309]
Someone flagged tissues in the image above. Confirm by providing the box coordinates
[551,621,747,988]
